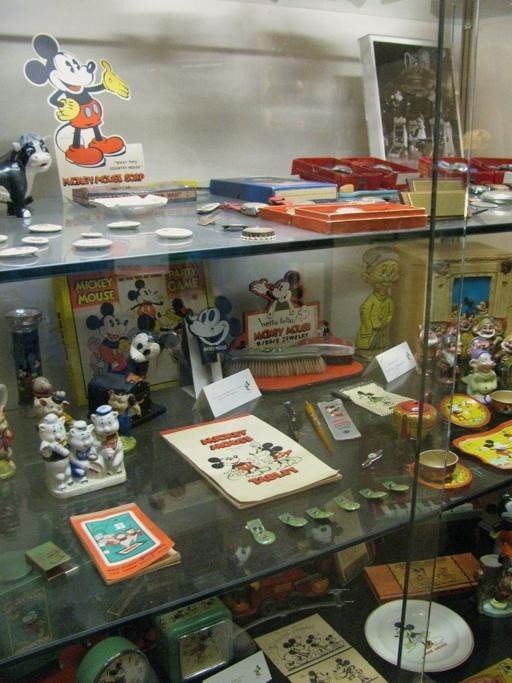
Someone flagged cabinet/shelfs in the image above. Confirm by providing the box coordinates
[1,176,512,682]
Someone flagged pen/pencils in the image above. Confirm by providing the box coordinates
[304,400,334,453]
[285,400,299,443]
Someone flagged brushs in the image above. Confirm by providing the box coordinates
[298,344,355,365]
[222,347,326,378]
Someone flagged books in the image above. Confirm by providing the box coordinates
[157,411,344,509]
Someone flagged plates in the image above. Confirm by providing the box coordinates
[481,187,512,204]
[406,459,472,493]
[437,391,491,431]
[0,212,196,260]
[362,598,476,675]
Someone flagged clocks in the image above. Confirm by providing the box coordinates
[75,636,151,682]
[152,596,239,682]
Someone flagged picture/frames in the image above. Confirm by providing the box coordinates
[356,31,464,162]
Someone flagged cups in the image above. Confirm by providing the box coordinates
[488,387,511,414]
[414,449,460,480]
